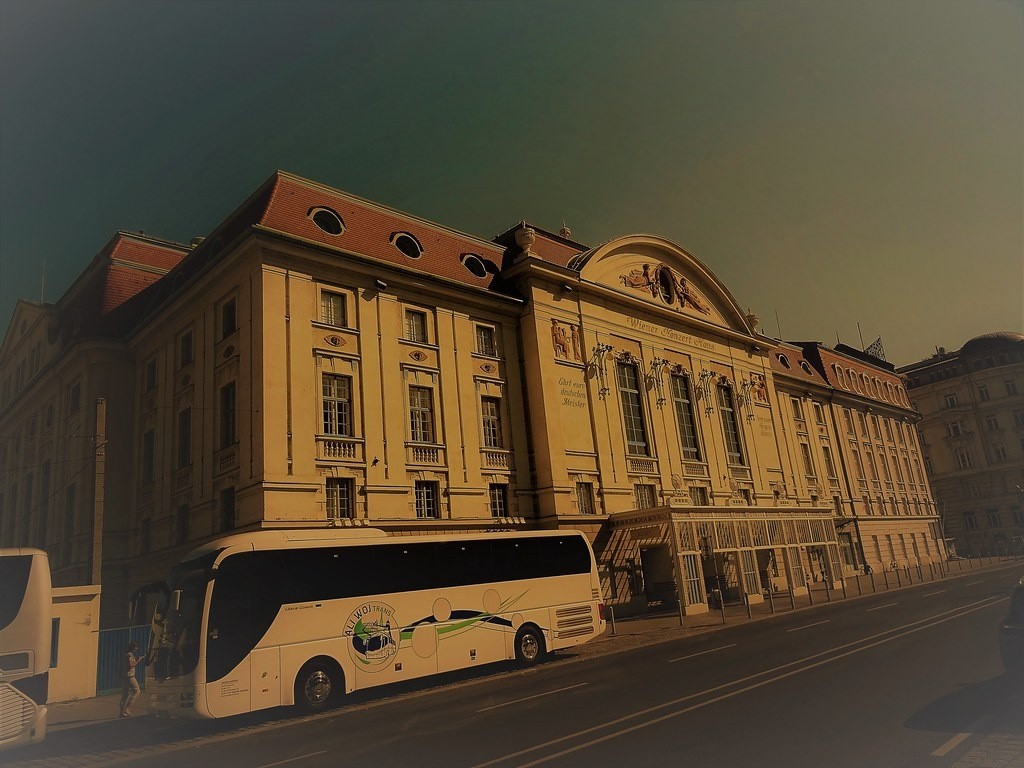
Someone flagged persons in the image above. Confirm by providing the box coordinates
[550,320,581,361]
[120,641,148,717]
[675,272,711,315]
[757,375,766,403]
[619,262,664,294]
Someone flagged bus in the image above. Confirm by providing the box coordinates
[0,548,51,745]
[127,529,609,718]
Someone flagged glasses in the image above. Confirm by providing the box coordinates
[134,649,138,651]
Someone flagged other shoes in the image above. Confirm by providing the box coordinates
[124,709,130,714]
[121,712,128,717]
